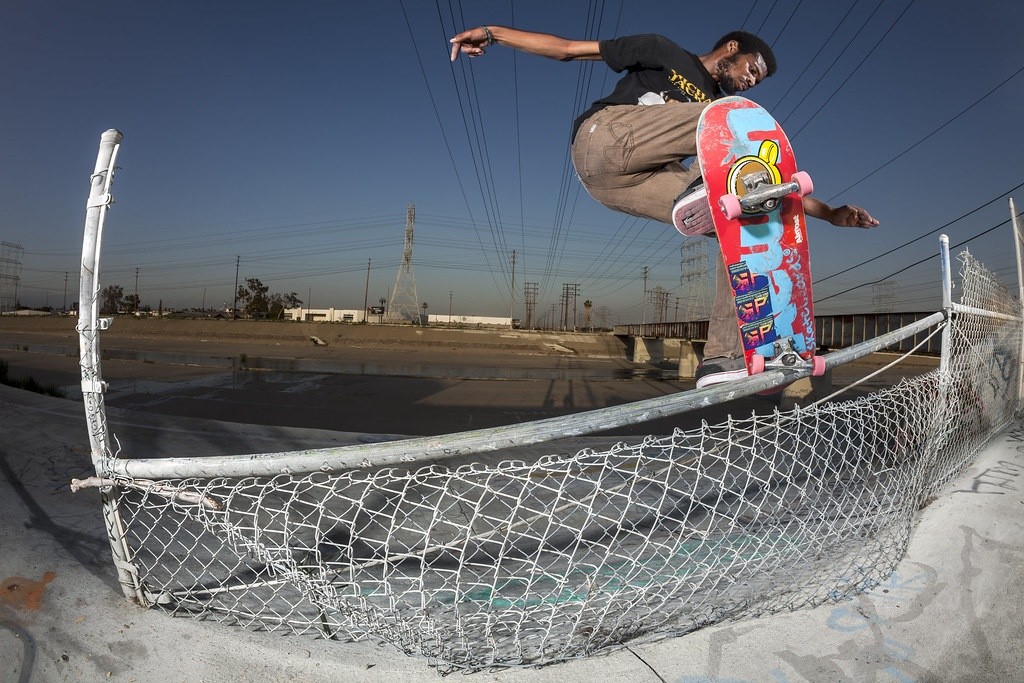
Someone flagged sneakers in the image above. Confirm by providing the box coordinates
[671,178,714,236]
[695,356,749,389]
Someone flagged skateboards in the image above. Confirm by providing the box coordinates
[694,95,816,373]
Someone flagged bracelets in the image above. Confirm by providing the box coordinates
[483,26,493,46]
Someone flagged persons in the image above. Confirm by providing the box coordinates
[448,22,879,393]
